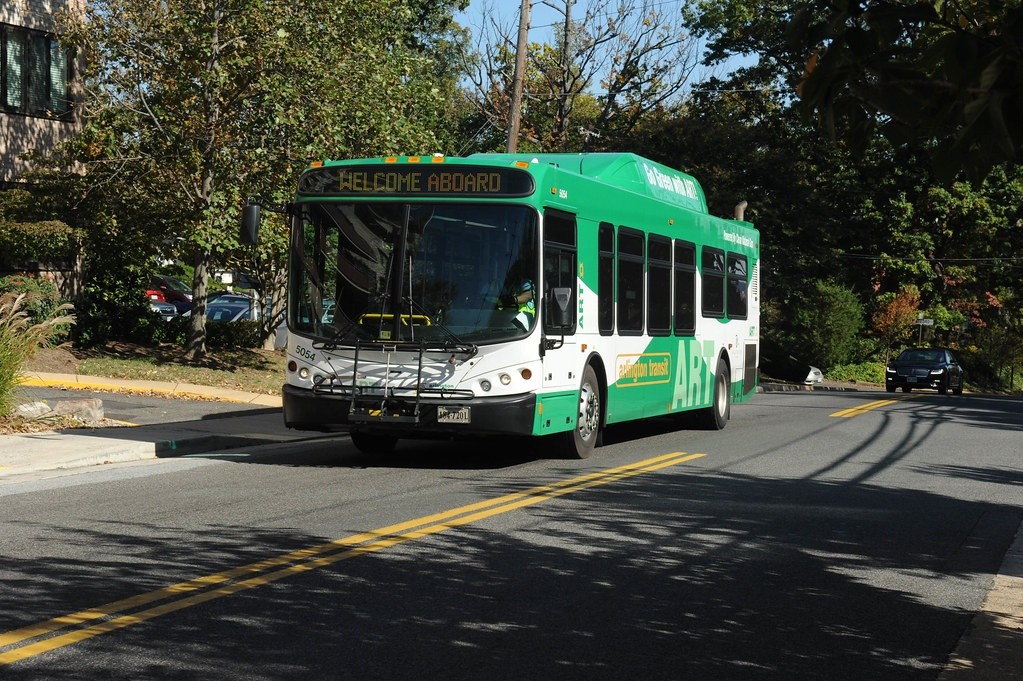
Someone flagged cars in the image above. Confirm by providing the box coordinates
[302,298,337,325]
[148,299,178,319]
[885,348,963,394]
[184,295,266,324]
[140,270,193,307]
[760,338,825,387]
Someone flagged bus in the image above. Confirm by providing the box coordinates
[239,151,761,458]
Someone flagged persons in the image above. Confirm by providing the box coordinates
[480,256,535,317]
